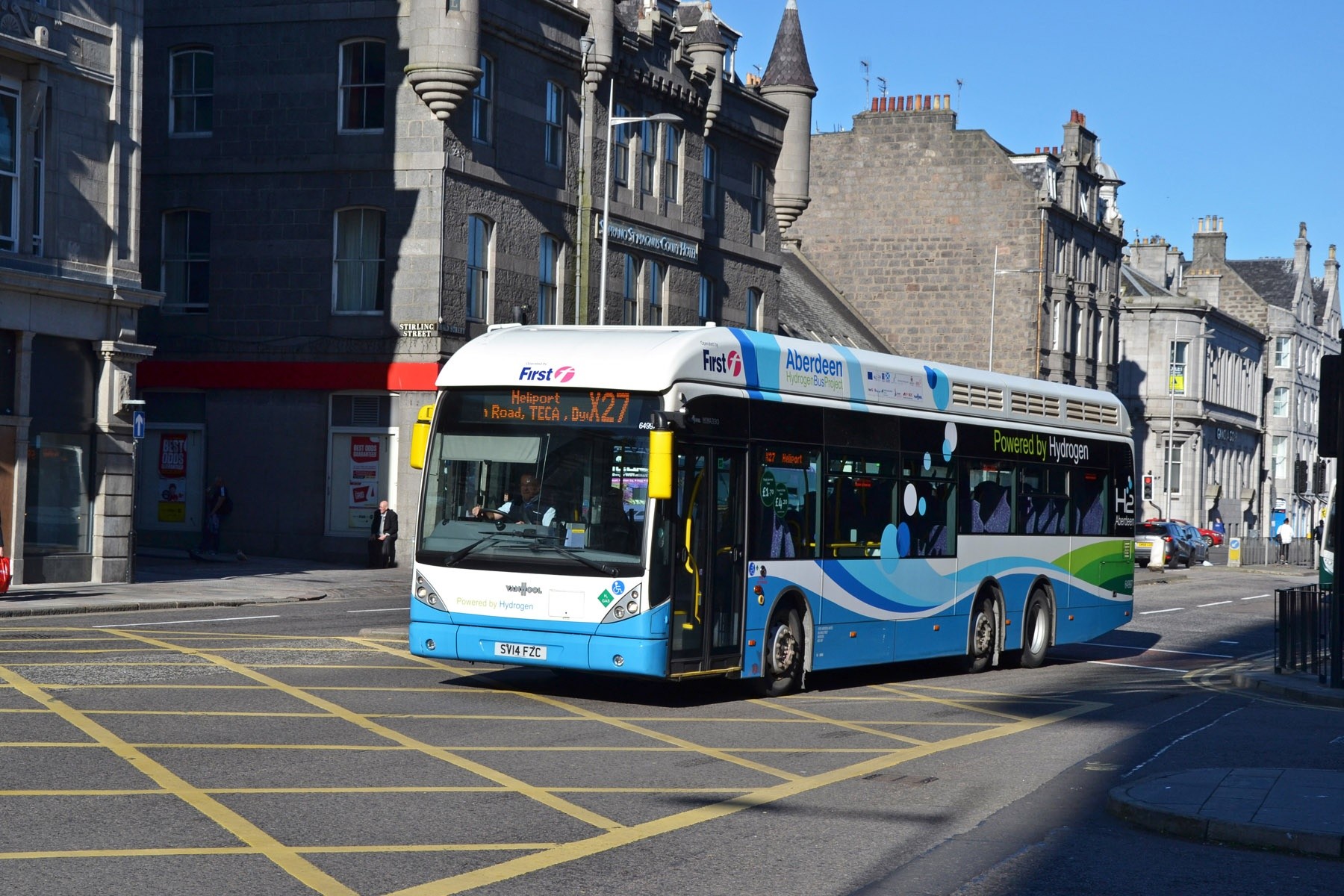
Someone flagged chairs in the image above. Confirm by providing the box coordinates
[761,502,795,559]
[916,481,1103,554]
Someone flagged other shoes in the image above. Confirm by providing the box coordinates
[382,564,390,568]
[1280,555,1284,562]
[195,550,203,555]
[1284,562,1288,565]
[369,562,376,568]
[208,550,219,556]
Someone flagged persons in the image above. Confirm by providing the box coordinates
[1276,518,1292,565]
[368,501,398,569]
[0,512,4,557]
[1314,520,1324,570]
[205,476,228,556]
[472,472,556,527]
[1213,518,1225,548]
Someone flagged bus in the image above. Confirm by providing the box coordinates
[409,324,1136,697]
[1319,478,1338,590]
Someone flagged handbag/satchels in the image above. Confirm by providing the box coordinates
[1275,534,1282,542]
[216,496,234,514]
[206,515,221,534]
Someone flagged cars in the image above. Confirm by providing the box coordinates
[1180,525,1210,563]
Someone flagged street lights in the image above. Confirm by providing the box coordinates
[1165,317,1216,524]
[598,80,684,328]
[988,246,1046,373]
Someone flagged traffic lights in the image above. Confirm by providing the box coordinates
[1141,476,1153,501]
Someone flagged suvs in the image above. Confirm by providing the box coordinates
[1135,522,1191,569]
[1145,519,1222,547]
